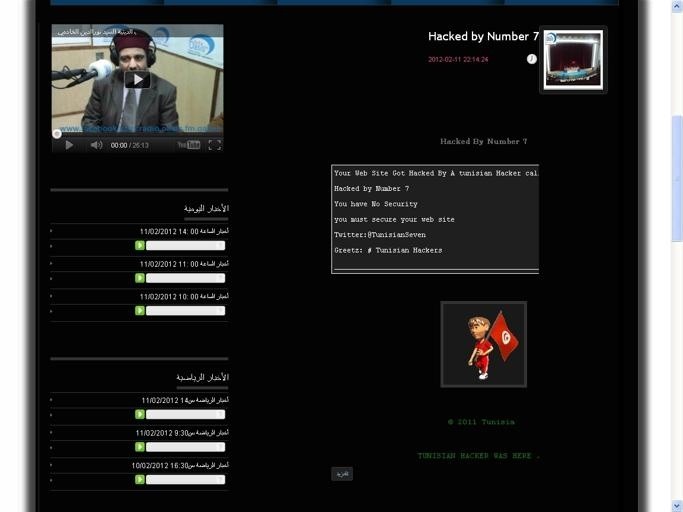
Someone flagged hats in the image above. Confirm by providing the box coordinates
[114,28,149,54]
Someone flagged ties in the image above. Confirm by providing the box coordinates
[120,88,137,132]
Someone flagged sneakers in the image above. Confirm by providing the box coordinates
[479,370,488,380]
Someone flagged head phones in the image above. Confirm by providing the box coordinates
[107,27,157,67]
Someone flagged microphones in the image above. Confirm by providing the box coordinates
[65,60,116,88]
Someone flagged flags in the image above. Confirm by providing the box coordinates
[490,312,519,363]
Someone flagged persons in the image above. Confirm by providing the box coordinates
[466,316,493,380]
[80,28,181,132]
